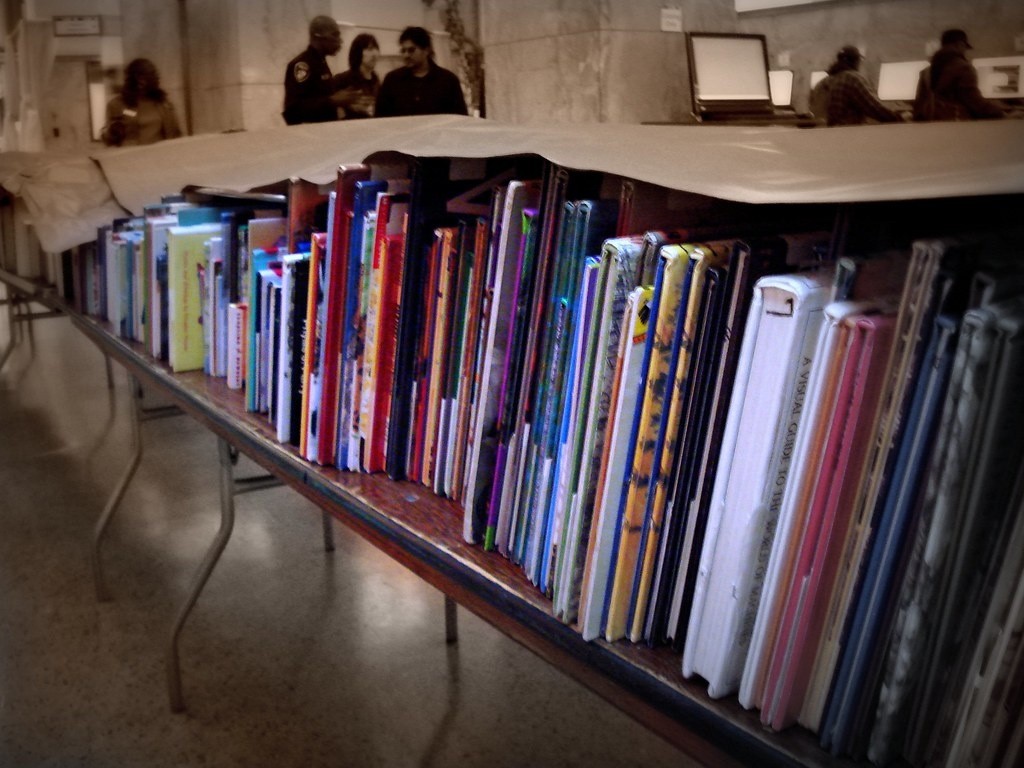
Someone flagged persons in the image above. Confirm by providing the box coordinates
[825,45,907,127]
[282,15,355,126]
[333,33,381,119]
[372,26,469,118]
[912,28,1004,124]
[105,58,182,148]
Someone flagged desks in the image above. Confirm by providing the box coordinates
[0,269,838,768]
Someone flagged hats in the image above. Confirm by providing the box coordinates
[941,29,972,50]
[838,46,866,60]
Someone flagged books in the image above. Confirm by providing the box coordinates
[22,164,1024,768]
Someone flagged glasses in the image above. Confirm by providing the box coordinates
[400,46,419,54]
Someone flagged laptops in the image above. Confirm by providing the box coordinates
[683,29,810,116]
